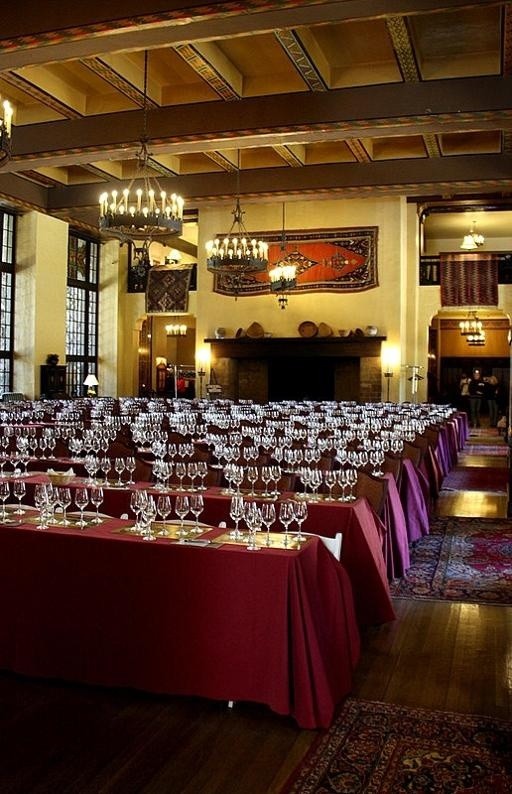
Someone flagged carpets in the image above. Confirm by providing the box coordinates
[262,428,510,794]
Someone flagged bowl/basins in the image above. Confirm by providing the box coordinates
[339,330,350,338]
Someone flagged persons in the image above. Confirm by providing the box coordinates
[484,375,499,426]
[458,372,471,408]
[467,368,485,427]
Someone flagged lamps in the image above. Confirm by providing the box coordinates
[97,48,186,273]
[461,220,485,251]
[205,146,269,296]
[83,375,101,397]
[381,346,398,401]
[195,348,209,401]
[458,308,484,346]
[269,195,296,307]
[2,99,14,140]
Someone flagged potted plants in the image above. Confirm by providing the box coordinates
[46,354,60,365]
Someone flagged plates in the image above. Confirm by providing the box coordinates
[366,325,378,337]
[299,322,317,338]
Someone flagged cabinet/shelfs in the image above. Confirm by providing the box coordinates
[40,365,67,399]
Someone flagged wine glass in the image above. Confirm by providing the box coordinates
[3,398,460,551]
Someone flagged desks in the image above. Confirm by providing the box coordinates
[0,399,468,720]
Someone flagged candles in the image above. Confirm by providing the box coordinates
[166,325,187,336]
